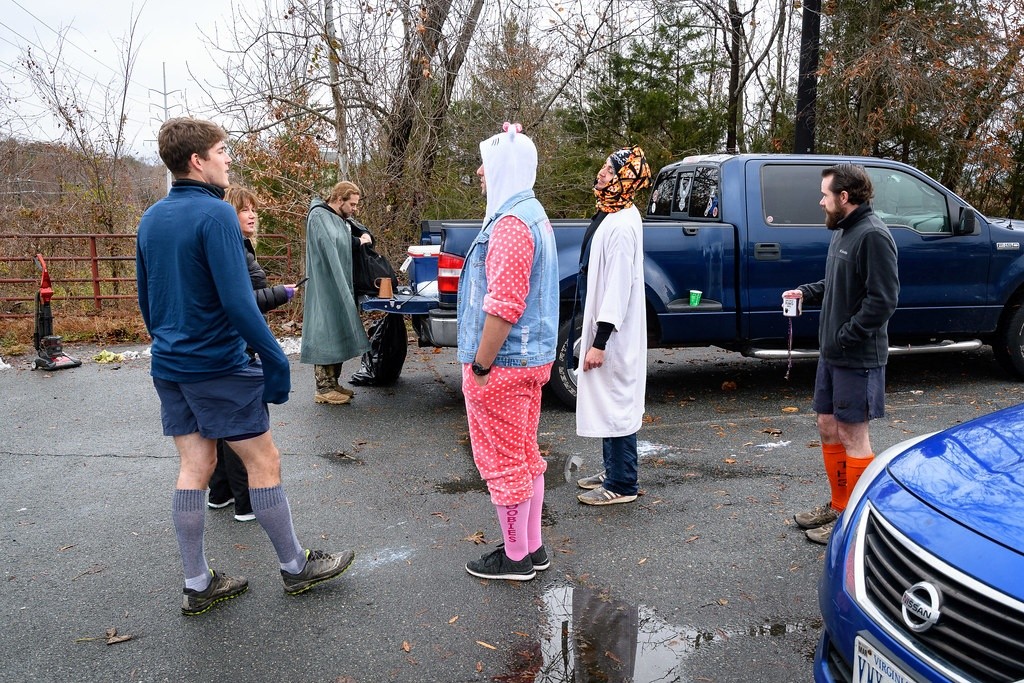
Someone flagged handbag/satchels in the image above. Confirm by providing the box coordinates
[348,313,408,387]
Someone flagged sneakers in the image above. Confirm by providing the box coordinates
[793,500,839,528]
[464,543,550,580]
[279,548,355,596]
[181,569,249,615]
[576,485,637,505]
[315,384,354,404]
[577,470,607,489]
[804,519,837,545]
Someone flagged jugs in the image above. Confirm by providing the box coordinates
[374,276,393,298]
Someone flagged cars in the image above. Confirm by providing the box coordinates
[814,402,1024,683]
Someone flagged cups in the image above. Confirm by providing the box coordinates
[689,290,702,305]
[782,294,803,316]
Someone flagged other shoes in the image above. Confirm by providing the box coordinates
[234,511,256,521]
[207,491,235,509]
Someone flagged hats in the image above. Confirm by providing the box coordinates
[609,149,633,174]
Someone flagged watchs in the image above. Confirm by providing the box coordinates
[472,359,490,377]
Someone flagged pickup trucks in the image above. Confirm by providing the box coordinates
[358,154,1023,410]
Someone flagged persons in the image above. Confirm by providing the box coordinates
[299,180,373,405]
[455,122,560,579]
[780,163,900,550]
[576,146,652,505]
[136,118,353,615]
[208,185,299,521]
